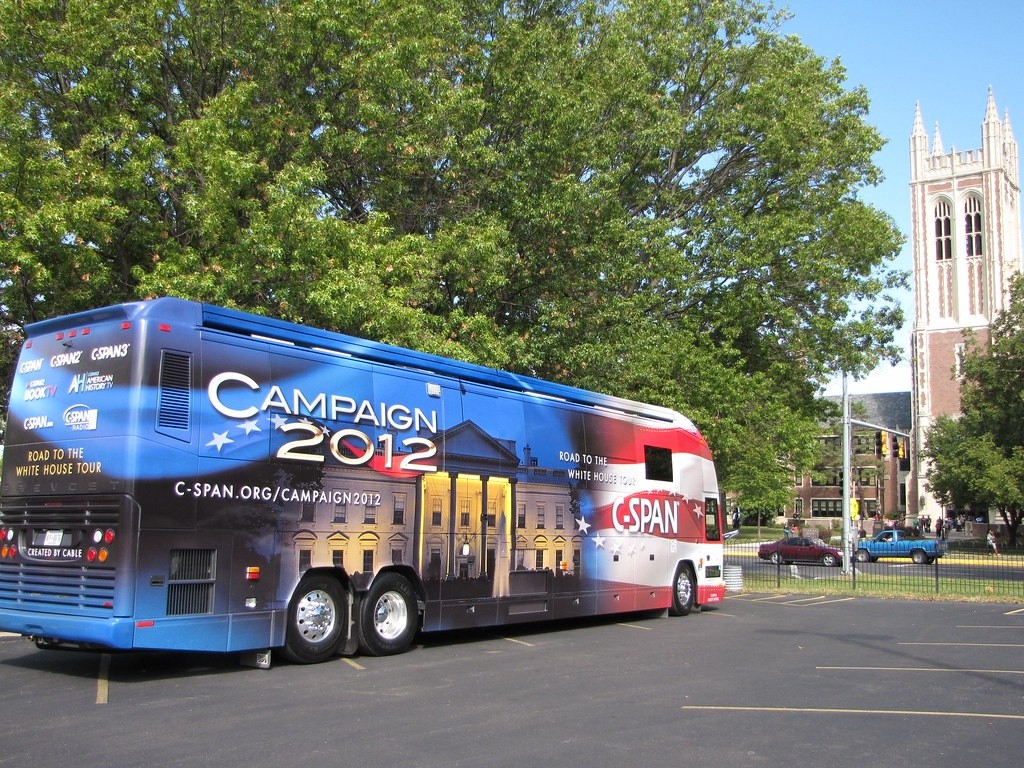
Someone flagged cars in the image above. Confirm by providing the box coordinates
[758,536,844,567]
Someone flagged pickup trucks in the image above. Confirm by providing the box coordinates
[856,530,947,564]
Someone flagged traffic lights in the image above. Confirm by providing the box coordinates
[875,430,886,457]
[892,436,903,459]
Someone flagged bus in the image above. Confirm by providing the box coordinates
[0,297,742,665]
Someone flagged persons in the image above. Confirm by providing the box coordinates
[791,524,797,537]
[935,516,954,538]
[955,514,967,532]
[883,515,898,530]
[784,522,789,537]
[882,534,893,541]
[874,513,880,521]
[795,512,799,519]
[976,515,985,523]
[987,530,997,555]
[732,507,741,530]
[913,515,931,536]
[858,526,866,538]
